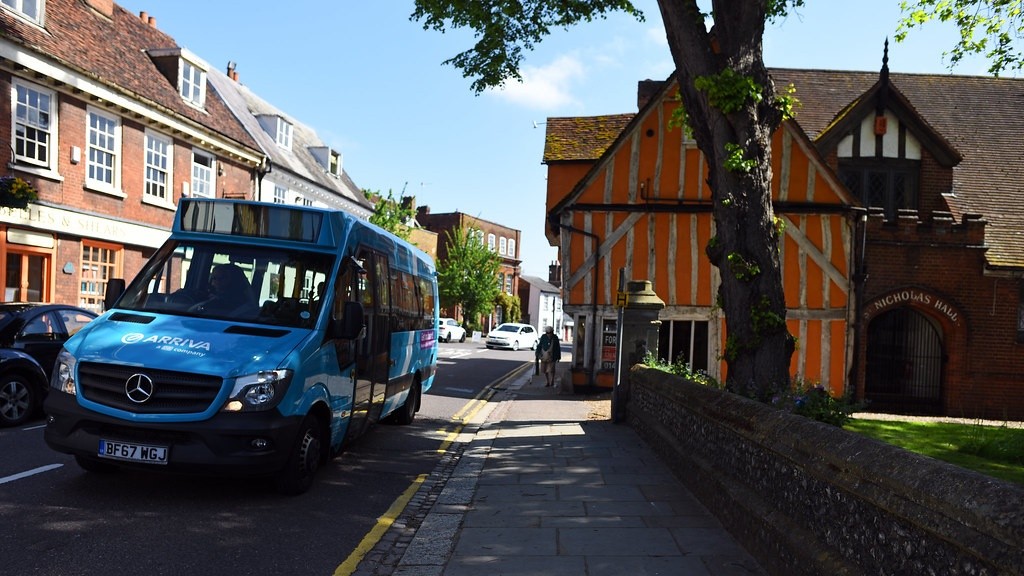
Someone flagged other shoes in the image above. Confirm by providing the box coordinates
[544,383,549,387]
[550,382,554,386]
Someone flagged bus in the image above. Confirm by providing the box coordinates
[48,198,440,498]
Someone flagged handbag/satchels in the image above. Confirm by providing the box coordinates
[541,340,553,362]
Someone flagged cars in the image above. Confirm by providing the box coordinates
[1,300,102,426]
[486,323,539,351]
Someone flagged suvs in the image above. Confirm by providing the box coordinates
[439,318,467,343]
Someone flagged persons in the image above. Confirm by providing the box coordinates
[175,265,243,318]
[535,326,561,387]
[309,282,324,309]
